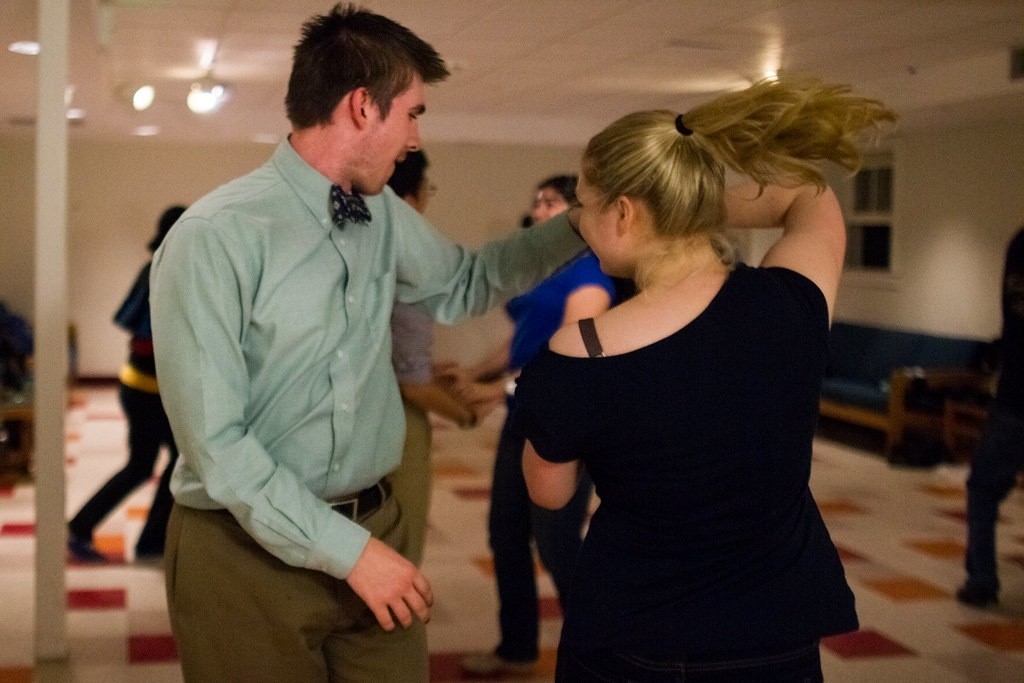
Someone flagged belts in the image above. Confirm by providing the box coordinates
[331,478,394,522]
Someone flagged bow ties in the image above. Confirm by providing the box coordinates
[331,184,373,227]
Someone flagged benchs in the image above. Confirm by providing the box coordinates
[820,320,996,459]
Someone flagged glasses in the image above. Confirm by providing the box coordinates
[416,185,436,198]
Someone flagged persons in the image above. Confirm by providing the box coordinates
[67,137,609,674]
[149,4,592,683]
[958,228,1024,605]
[522,75,901,682]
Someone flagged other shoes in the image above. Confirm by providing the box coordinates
[68,531,102,561]
[463,650,535,676]
[958,587,998,607]
